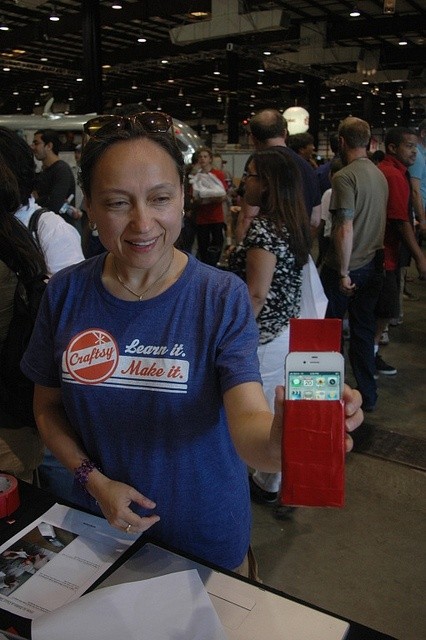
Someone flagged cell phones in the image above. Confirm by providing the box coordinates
[284,350,345,401]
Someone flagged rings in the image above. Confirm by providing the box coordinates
[124,523,133,534]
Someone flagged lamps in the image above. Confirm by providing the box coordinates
[283,98,310,136]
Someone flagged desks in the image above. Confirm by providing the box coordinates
[0,471,399,639]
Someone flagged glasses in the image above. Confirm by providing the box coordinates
[243,172,257,180]
[81,112,177,155]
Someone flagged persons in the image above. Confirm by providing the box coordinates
[64,142,89,243]
[0,164,47,429]
[187,147,229,268]
[31,127,76,225]
[19,113,365,587]
[328,117,390,413]
[235,107,322,270]
[2,126,86,282]
[408,119,426,248]
[286,133,322,171]
[318,129,341,172]
[373,127,425,376]
[219,149,313,519]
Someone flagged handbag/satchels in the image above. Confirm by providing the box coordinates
[298,253,329,320]
[31,440,92,512]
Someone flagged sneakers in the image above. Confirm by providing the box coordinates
[362,394,378,410]
[274,505,296,518]
[390,314,405,325]
[251,481,277,502]
[379,330,388,344]
[374,355,397,374]
[402,291,419,300]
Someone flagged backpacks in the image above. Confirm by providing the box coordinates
[0,207,51,403]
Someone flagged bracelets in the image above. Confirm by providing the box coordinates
[72,459,96,496]
[341,273,349,278]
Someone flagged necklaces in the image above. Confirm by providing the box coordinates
[108,251,177,303]
